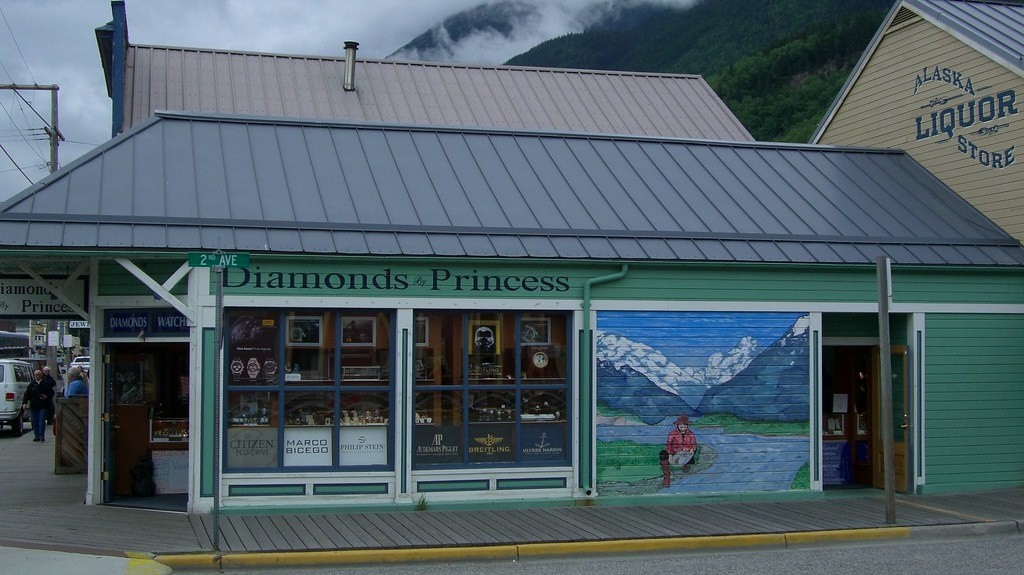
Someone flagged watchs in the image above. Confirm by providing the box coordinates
[480,402,513,421]
[245,356,261,381]
[534,399,562,420]
[230,355,244,383]
[263,354,277,383]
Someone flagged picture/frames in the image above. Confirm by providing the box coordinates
[341,315,376,347]
[514,317,551,347]
[415,317,429,346]
[468,319,501,356]
[284,316,324,347]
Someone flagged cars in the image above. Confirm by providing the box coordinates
[70,355,90,375]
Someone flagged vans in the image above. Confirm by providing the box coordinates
[0,358,36,435]
[14,356,65,398]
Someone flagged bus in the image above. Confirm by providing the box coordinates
[0,331,29,360]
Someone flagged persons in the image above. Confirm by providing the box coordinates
[63,367,89,400]
[77,365,88,381]
[41,365,58,425]
[22,369,56,441]
[344,319,362,343]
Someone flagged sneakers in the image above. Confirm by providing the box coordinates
[39,438,45,443]
[33,438,40,442]
